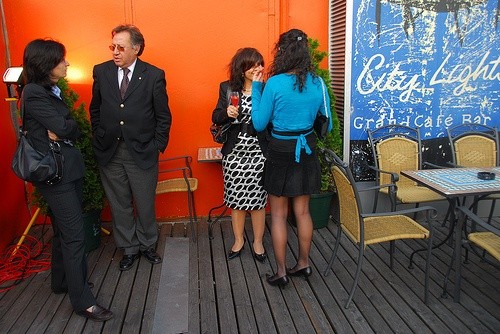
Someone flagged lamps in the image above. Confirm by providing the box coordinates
[2,65,24,86]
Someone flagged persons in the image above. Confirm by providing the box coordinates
[251,29,332,289]
[89,25,172,271]
[16,39,114,321]
[212,48,268,263]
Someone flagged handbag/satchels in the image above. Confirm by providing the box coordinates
[210,80,234,143]
[313,76,330,141]
[11,130,63,187]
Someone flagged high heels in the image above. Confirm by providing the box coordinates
[252,240,266,264]
[267,275,289,290]
[227,237,246,259]
[287,266,313,281]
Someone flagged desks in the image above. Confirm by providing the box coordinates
[400,166,500,298]
[196,146,228,239]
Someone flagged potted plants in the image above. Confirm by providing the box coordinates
[290,38,342,229]
[32,79,103,252]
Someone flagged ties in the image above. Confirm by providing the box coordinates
[119,67,131,100]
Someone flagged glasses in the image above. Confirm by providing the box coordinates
[109,44,124,52]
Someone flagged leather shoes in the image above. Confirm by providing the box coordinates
[120,253,141,271]
[140,249,161,263]
[76,304,114,322]
[53,281,94,294]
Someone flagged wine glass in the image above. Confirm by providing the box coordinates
[230,91,242,124]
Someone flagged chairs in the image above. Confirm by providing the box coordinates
[455,205,500,303]
[443,123,500,228]
[319,146,438,309]
[156,155,198,241]
[365,125,453,253]
